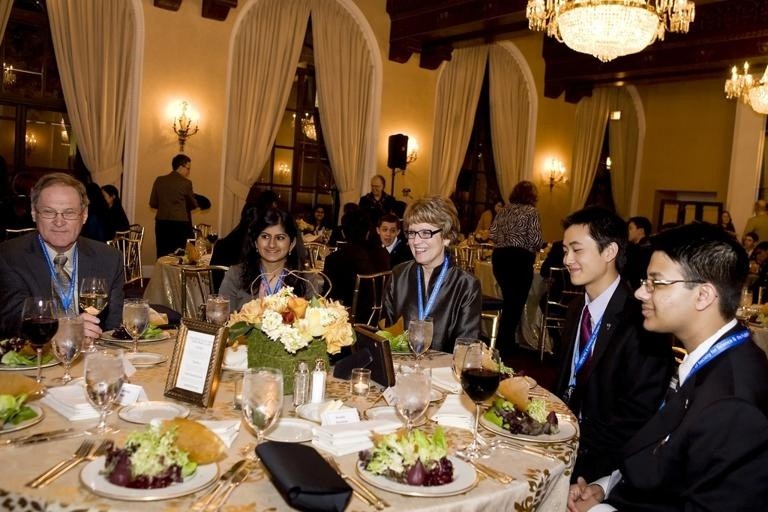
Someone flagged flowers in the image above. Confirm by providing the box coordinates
[226,288,351,351]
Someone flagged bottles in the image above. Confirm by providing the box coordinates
[292,360,327,408]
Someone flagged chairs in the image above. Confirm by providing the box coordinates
[107,224,582,386]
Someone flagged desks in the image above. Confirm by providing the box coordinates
[736,303,768,359]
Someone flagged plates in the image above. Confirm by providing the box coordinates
[294,401,361,424]
[0,327,191,433]
[264,417,322,444]
[424,366,463,395]
[354,452,480,498]
[363,405,430,428]
[479,409,576,442]
[79,444,221,502]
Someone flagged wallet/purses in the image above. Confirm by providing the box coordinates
[255,441,353,511]
[148,302,183,329]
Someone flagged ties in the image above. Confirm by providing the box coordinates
[576,306,594,382]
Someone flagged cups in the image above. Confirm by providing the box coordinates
[233,371,246,412]
[206,294,231,327]
[451,338,485,398]
[349,367,372,400]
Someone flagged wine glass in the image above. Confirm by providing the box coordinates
[241,366,284,461]
[20,277,150,437]
[459,346,500,458]
[396,315,501,460]
[207,229,218,257]
[408,314,434,367]
[396,361,433,430]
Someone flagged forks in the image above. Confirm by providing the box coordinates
[326,455,392,508]
[23,437,115,490]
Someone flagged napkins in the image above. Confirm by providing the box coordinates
[426,364,466,393]
[431,394,477,430]
[292,397,358,424]
[39,384,100,424]
[310,417,395,455]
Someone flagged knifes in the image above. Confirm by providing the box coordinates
[185,459,258,512]
[0,428,85,449]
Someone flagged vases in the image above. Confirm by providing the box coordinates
[245,327,331,392]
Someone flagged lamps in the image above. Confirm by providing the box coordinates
[542,159,567,192]
[173,101,199,153]
[302,114,317,140]
[526,0,696,63]
[402,149,417,175]
[610,88,621,121]
[3,63,16,88]
[724,61,768,115]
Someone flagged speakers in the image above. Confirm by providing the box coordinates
[387,134,408,170]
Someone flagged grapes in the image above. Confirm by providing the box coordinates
[423,456,454,486]
[503,412,522,435]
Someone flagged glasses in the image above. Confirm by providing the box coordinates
[402,227,444,241]
[32,203,86,221]
[639,276,706,294]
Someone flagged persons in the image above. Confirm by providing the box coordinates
[474,199,503,233]
[149,154,199,259]
[488,181,544,348]
[554,206,675,482]
[261,194,325,226]
[81,182,110,243]
[322,176,412,323]
[220,209,320,316]
[12,174,37,216]
[628,200,767,303]
[0,173,124,345]
[566,222,767,512]
[375,193,483,355]
[100,185,130,251]
